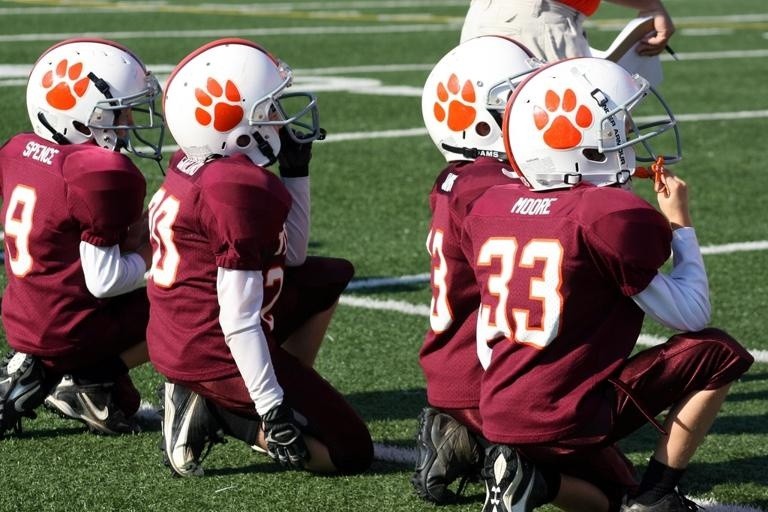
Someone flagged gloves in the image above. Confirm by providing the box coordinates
[277,125,312,177]
[261,404,311,471]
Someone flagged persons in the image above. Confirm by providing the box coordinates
[465,54,755,511]
[411,33,642,511]
[0,37,175,437]
[139,37,379,478]
[458,0,676,70]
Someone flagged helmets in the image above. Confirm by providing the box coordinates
[25,37,161,149]
[161,38,294,167]
[420,34,650,192]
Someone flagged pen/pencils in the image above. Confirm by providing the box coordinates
[664,44,682,64]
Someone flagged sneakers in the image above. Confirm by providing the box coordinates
[480,445,539,512]
[157,382,216,479]
[0,350,142,436]
[411,406,475,506]
[620,493,699,512]
